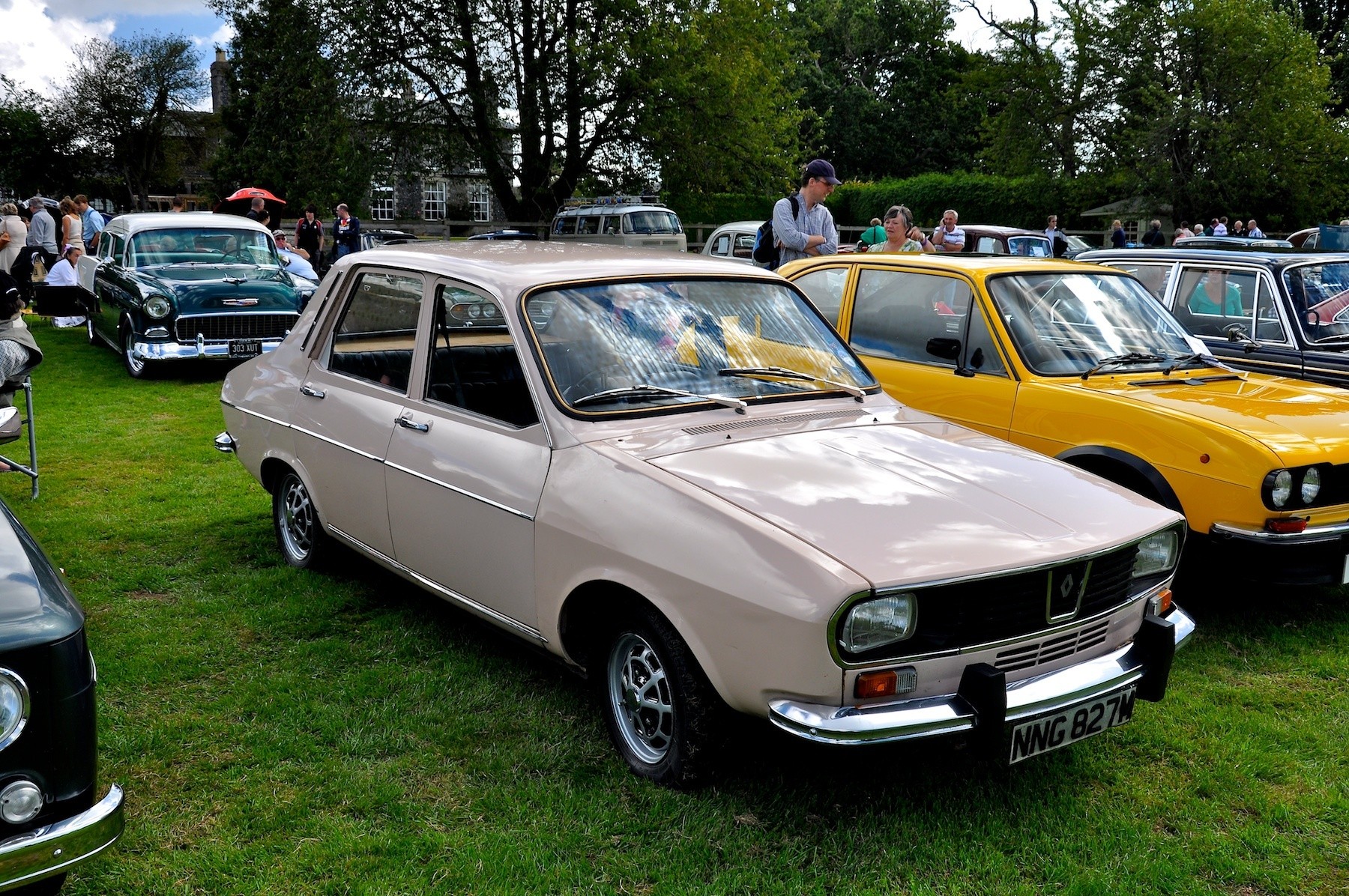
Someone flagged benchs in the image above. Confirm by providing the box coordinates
[332,342,596,427]
[823,305,946,347]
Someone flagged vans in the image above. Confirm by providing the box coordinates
[550,194,687,253]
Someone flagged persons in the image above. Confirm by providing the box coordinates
[861,204,965,309]
[1018,214,1261,317]
[772,159,843,267]
[0,195,360,472]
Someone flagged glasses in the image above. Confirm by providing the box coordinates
[813,177,832,186]
[276,237,286,241]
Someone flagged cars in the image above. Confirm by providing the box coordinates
[959,224,1091,301]
[315,229,557,332]
[699,221,870,273]
[673,251,1349,586]
[0,497,126,896]
[1061,224,1349,391]
[214,237,1197,782]
[468,230,541,242]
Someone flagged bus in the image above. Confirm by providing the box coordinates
[132,194,212,213]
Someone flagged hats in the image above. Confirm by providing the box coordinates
[0,271,20,302]
[807,159,843,185]
[273,230,286,237]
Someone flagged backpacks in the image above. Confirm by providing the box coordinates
[89,210,112,226]
[751,196,800,271]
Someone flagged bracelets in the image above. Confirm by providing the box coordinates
[942,242,946,246]
[920,238,927,246]
[940,227,944,230]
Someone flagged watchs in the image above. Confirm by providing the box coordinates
[917,232,925,241]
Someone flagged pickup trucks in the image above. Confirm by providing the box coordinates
[75,211,319,378]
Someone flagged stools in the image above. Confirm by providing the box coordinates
[0,376,39,500]
